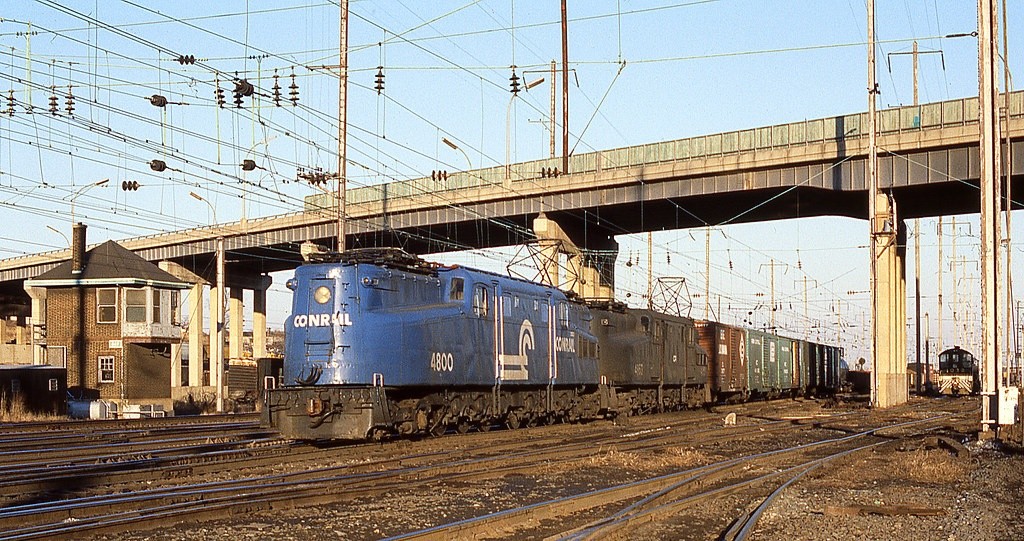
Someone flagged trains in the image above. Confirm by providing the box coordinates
[264,244,846,444]
[938,345,981,397]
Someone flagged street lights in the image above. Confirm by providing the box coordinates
[71,177,109,228]
[506,75,544,178]
[189,191,224,413]
